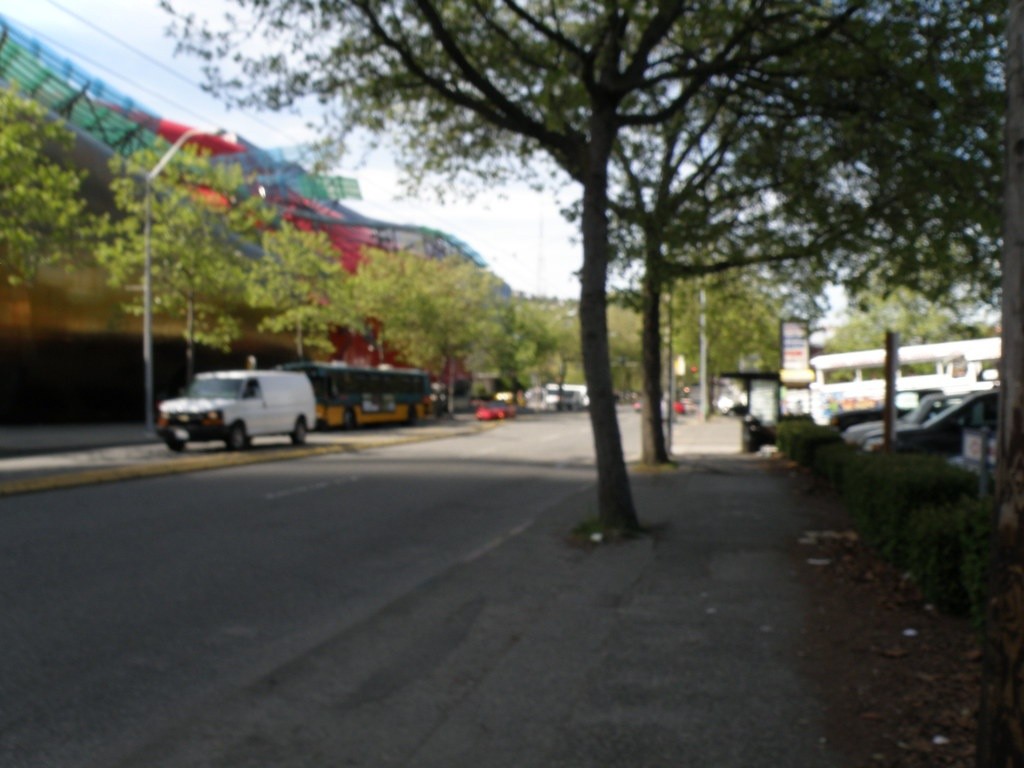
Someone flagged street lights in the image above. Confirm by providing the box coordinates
[142,128,233,433]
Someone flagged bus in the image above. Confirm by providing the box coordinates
[808,336,1002,428]
[272,360,430,432]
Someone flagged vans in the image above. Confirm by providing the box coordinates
[155,369,316,453]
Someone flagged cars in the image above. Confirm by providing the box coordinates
[657,382,751,423]
[829,385,1002,474]
[471,382,639,421]
